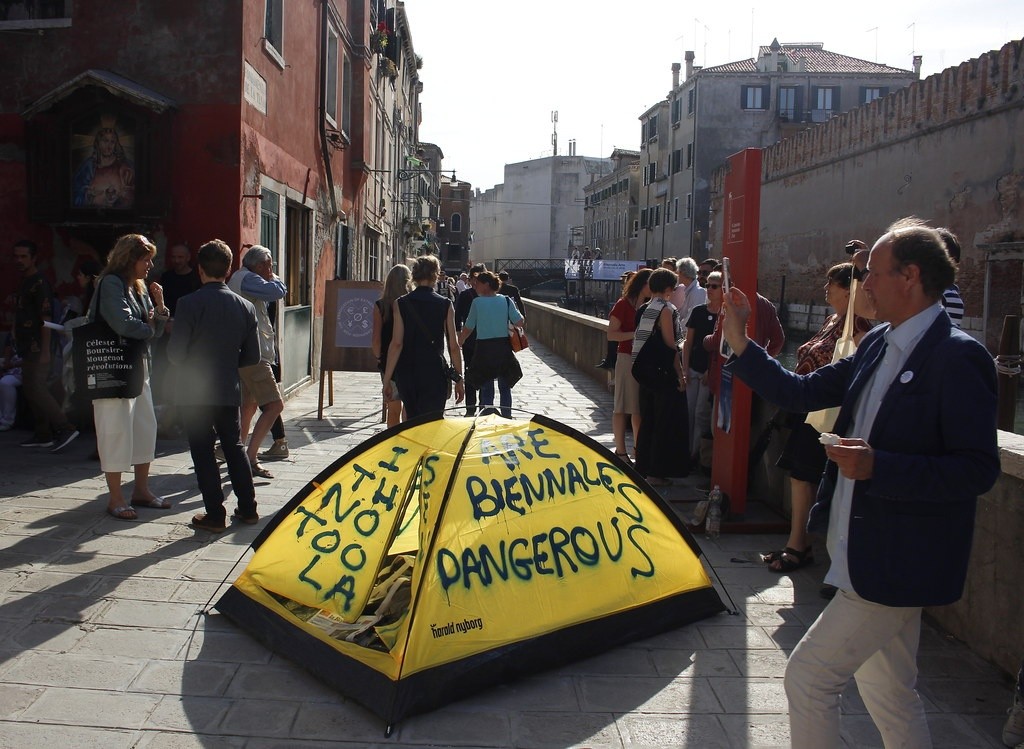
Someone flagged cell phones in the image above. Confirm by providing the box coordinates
[723,258,730,295]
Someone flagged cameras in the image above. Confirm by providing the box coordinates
[845,243,860,254]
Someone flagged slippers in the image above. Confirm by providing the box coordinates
[132,498,171,509]
[107,503,140,518]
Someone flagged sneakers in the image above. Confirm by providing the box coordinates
[213,444,228,463]
[21,434,54,447]
[49,430,79,451]
[191,512,226,533]
[233,508,260,524]
[259,441,290,458]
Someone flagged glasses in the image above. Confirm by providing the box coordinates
[705,284,722,289]
[696,270,709,275]
[439,274,446,277]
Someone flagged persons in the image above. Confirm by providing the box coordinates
[0,240,200,460]
[455,264,494,417]
[166,240,261,532]
[820,228,964,598]
[764,264,873,572]
[458,272,524,419]
[572,247,579,259]
[85,234,172,520]
[582,247,591,259]
[383,256,464,419]
[722,216,1000,749]
[594,248,603,260]
[214,245,289,479]
[372,264,416,428]
[434,271,526,330]
[595,258,785,513]
[1002,657,1024,749]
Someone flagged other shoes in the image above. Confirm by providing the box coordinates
[597,361,616,369]
[821,587,839,600]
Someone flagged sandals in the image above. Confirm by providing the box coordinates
[767,549,816,573]
[614,451,635,467]
[251,461,275,478]
[762,552,783,562]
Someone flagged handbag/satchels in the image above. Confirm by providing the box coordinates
[509,324,529,353]
[805,264,859,434]
[631,302,678,394]
[71,274,145,398]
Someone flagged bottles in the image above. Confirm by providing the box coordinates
[705,484,723,539]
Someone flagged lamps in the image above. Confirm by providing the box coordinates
[398,168,458,187]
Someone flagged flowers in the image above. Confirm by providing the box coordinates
[370,18,388,53]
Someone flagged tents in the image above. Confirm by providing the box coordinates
[200,405,740,739]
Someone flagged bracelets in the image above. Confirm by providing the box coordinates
[678,376,685,381]
